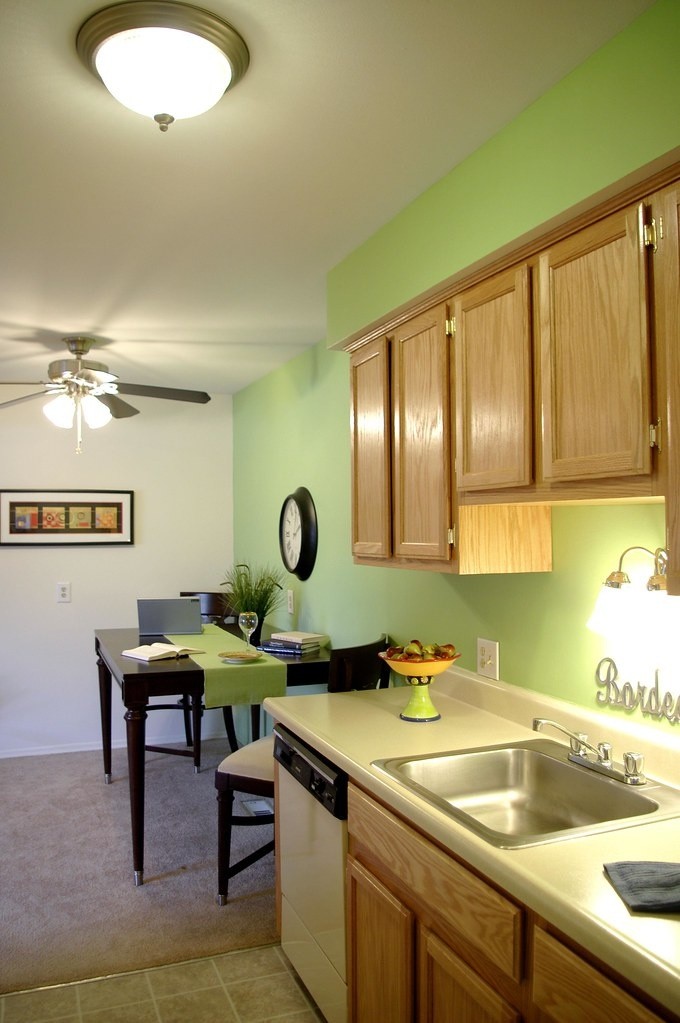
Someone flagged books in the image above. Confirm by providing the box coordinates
[256,631,324,654]
[121,642,205,660]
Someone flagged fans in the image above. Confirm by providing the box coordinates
[0,369,211,419]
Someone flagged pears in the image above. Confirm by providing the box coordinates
[387,639,455,660]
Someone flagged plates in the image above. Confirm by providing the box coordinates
[218,651,262,663]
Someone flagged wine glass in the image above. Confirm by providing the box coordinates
[239,612,258,652]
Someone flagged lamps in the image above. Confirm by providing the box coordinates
[584,546,668,631]
[76,0,250,132]
[46,336,111,451]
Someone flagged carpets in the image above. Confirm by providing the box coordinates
[0,737,280,995]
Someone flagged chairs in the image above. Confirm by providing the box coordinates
[146,592,238,774]
[214,634,391,905]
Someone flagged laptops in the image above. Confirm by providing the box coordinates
[137,597,204,635]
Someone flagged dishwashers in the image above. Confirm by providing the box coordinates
[270,722,350,1023]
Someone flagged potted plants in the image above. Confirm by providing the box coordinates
[217,562,290,647]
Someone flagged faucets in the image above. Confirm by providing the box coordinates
[532,717,647,785]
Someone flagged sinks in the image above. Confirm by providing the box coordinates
[371,738,680,848]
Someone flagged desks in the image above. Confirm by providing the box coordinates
[95,623,332,887]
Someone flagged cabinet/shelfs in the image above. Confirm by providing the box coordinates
[341,161,680,595]
[344,775,680,1022]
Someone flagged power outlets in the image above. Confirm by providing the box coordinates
[476,638,499,681]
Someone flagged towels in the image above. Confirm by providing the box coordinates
[602,859,680,914]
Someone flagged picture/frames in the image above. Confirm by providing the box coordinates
[0,489,135,546]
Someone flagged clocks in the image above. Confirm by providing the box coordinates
[279,486,318,581]
